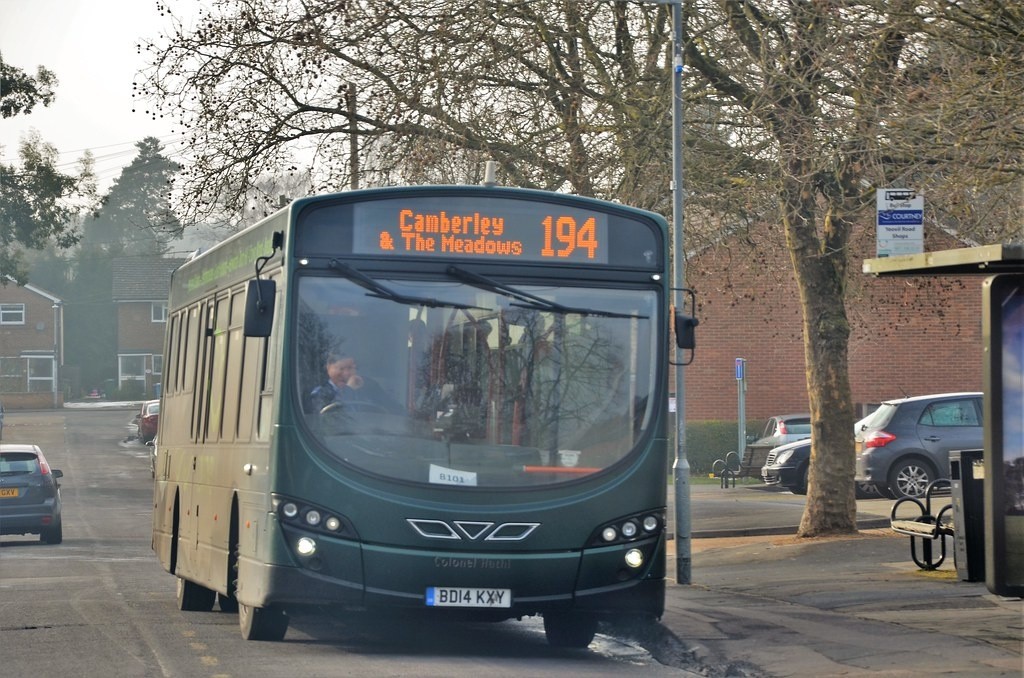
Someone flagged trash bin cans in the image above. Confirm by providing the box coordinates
[948,449,989,583]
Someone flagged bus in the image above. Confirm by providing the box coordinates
[156,182,700,653]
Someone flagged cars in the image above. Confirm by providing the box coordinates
[0,443,65,543]
[138,400,161,442]
[757,412,883,500]
[862,392,986,500]
[761,411,816,441]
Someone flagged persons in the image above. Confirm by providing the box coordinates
[307,350,366,414]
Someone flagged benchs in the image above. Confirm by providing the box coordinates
[712,451,743,489]
[890,477,956,570]
